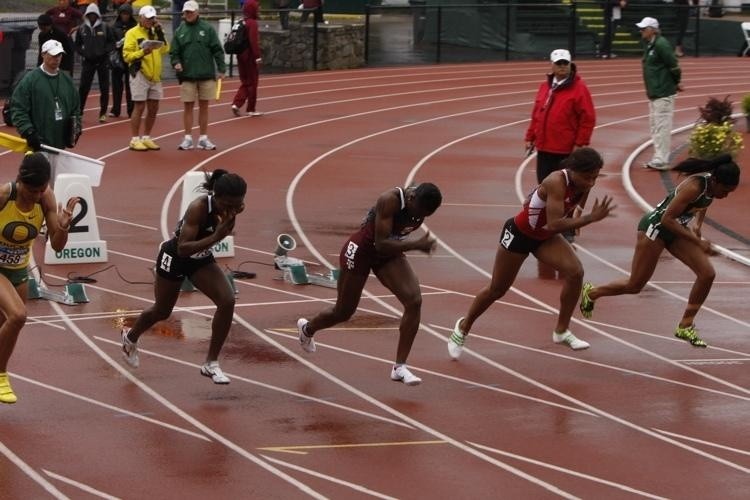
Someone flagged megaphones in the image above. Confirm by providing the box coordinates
[275,233,296,255]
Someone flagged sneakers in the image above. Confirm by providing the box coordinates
[230,104,242,116]
[140,139,161,151]
[642,159,671,171]
[446,317,466,361]
[246,111,263,117]
[296,317,317,354]
[199,360,231,385]
[389,362,421,387]
[130,140,147,152]
[198,139,216,150]
[551,327,589,353]
[178,140,194,150]
[120,326,139,368]
[0,372,17,403]
[97,112,116,122]
[578,280,595,318]
[674,323,706,348]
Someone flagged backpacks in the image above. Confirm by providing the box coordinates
[223,18,249,54]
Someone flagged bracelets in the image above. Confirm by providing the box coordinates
[581,212,592,224]
[56,222,70,234]
[573,205,583,219]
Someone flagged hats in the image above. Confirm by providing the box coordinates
[139,4,156,20]
[182,0,200,13]
[118,3,133,16]
[550,49,573,64]
[636,16,658,31]
[41,38,66,56]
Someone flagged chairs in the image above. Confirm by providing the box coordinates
[741,21,750,57]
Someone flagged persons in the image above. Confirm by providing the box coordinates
[240,0,245,10]
[36,0,152,123]
[296,182,441,385]
[168,0,226,150]
[231,0,264,117]
[664,0,689,58]
[172,0,189,33]
[578,153,740,349]
[447,146,618,361]
[122,5,172,150]
[121,168,248,383]
[11,39,81,188]
[601,0,627,58]
[278,0,289,29]
[523,48,597,253]
[636,16,684,171]
[302,0,326,25]
[0,147,80,402]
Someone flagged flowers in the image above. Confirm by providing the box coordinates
[689,121,744,160]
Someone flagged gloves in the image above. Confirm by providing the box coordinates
[25,130,46,152]
